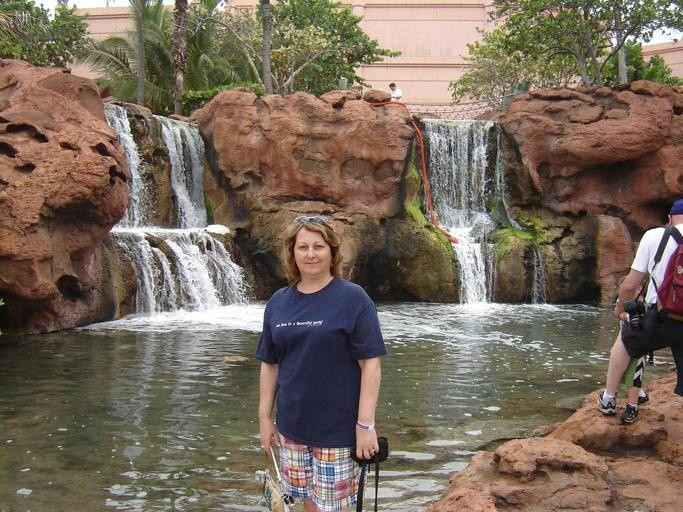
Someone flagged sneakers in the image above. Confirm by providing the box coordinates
[598,390,616,416]
[620,404,638,424]
[637,392,649,405]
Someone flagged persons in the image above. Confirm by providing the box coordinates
[250,212,384,512]
[512,67,530,97]
[613,267,650,426]
[387,82,402,104]
[596,197,682,416]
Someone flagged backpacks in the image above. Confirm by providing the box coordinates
[658,223,683,322]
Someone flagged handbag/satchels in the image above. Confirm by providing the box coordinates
[262,474,288,512]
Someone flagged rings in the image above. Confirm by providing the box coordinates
[369,450,374,453]
[259,445,264,450]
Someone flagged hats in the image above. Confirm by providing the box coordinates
[670,198,683,214]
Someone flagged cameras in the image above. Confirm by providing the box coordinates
[624,301,645,330]
[351,437,389,464]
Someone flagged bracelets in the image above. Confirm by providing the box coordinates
[354,421,376,432]
[615,297,624,309]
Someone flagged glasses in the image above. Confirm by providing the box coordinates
[294,216,327,225]
